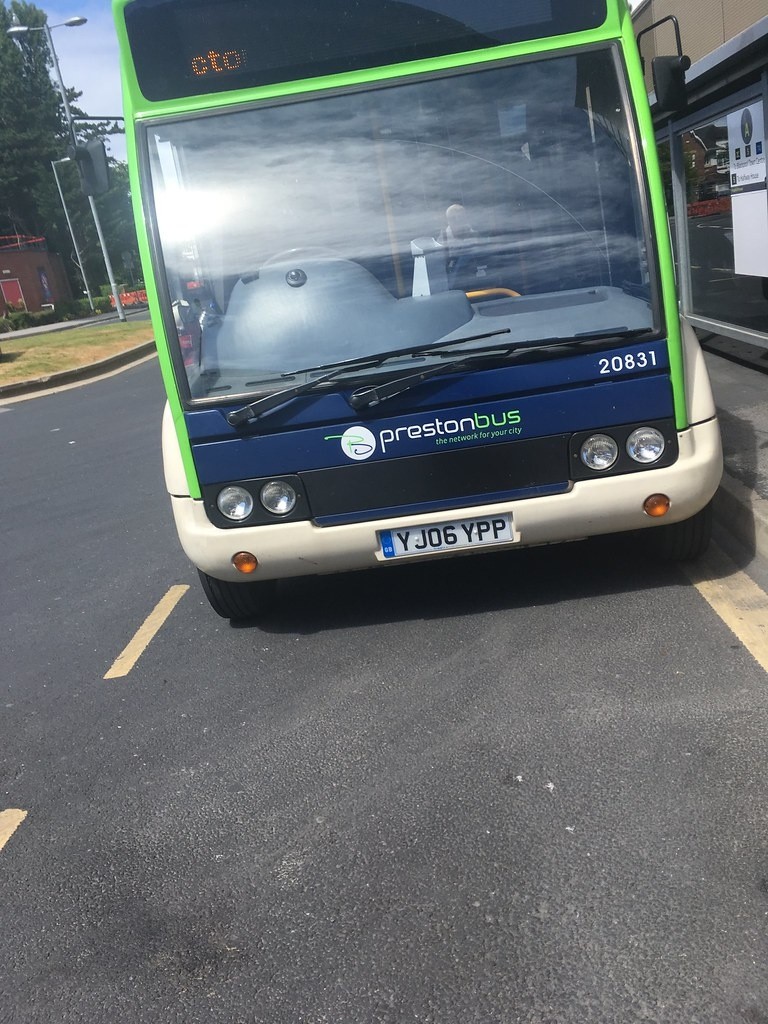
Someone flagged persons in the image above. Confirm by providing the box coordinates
[436,205,480,253]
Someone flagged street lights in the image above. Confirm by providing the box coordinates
[51,157,95,311]
[6,14,129,324]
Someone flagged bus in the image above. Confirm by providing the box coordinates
[64,0,724,622]
